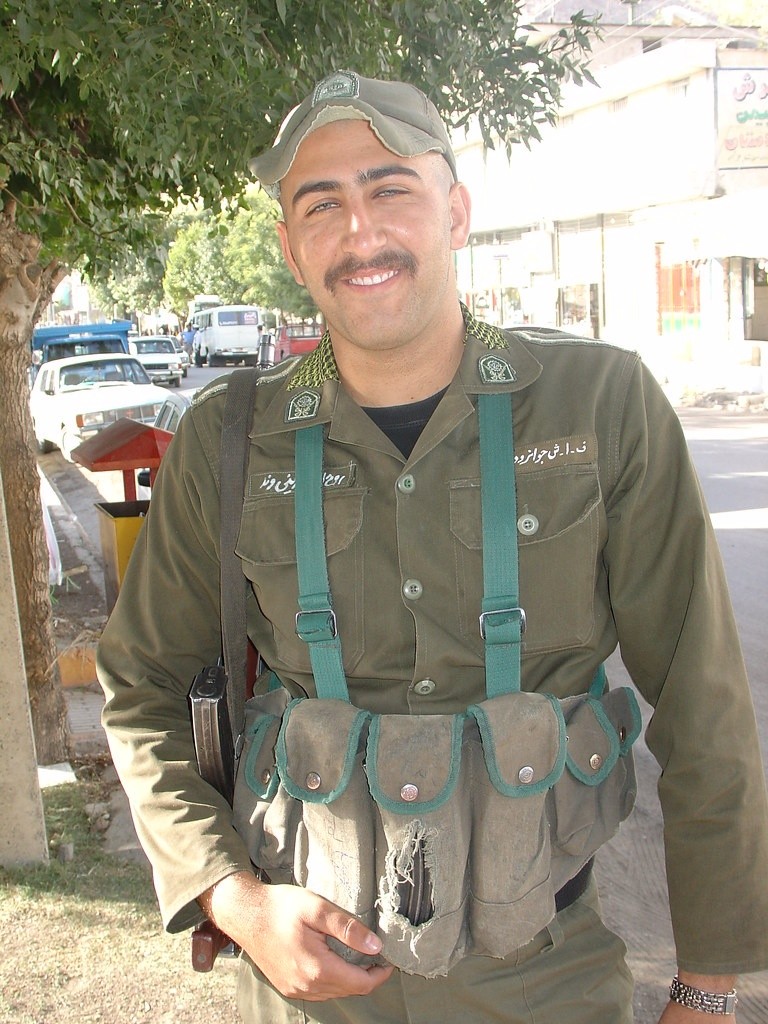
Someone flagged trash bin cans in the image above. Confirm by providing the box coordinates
[69,417,175,623]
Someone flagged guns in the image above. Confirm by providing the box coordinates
[184,664,242,973]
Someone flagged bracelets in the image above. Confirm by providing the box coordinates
[669,975,738,1015]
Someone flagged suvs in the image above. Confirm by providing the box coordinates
[27,292,225,390]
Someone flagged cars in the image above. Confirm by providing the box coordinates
[26,351,175,463]
[138,386,205,500]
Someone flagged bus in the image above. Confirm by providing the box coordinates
[181,303,264,368]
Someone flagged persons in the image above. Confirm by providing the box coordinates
[192,324,203,367]
[95,69,767,1023]
[257,325,265,351]
[180,325,206,364]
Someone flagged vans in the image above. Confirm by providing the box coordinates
[255,322,324,369]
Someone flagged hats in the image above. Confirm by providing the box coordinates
[249,71,457,200]
